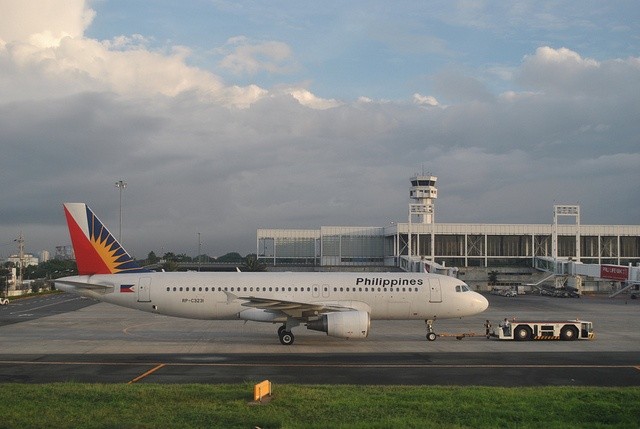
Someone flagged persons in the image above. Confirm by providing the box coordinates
[498,317,510,334]
[483,319,492,337]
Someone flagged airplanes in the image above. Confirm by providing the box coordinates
[34,202,490,345]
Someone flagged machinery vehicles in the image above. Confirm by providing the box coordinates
[489,315,596,340]
